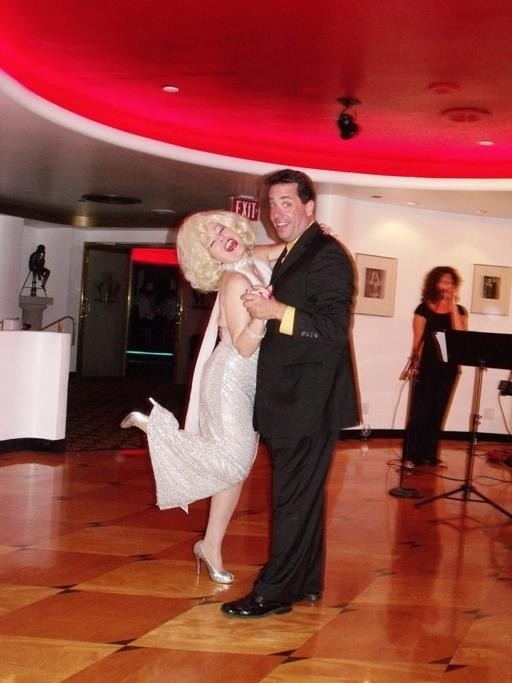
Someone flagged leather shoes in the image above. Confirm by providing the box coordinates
[220,592,292,618]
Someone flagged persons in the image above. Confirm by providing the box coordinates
[120,208,334,583]
[219,169,363,618]
[29,244,50,290]
[401,265,470,470]
[369,271,381,297]
[484,277,496,299]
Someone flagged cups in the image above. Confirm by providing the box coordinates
[3,318,24,330]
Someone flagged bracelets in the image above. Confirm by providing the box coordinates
[245,325,267,342]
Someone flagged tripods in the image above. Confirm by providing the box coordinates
[415,371,512,521]
[19,269,50,301]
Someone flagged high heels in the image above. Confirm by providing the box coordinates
[120,397,157,434]
[194,540,234,583]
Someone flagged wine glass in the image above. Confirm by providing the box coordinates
[361,425,370,440]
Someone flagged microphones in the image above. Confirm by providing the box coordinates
[439,288,461,302]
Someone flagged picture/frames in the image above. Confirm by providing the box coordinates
[468,262,511,318]
[352,249,398,318]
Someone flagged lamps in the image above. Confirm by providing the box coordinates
[335,92,363,142]
